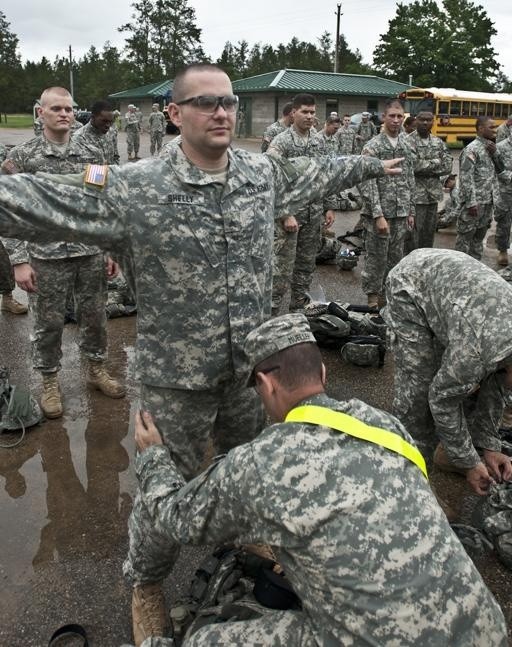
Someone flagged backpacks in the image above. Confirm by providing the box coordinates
[304,301,349,349]
[342,305,387,367]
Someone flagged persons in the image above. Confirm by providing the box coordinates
[2,64,406,647]
[262,93,510,311]
[135,312,510,646]
[0,85,126,419]
[70,102,167,165]
[382,247,512,493]
[0,143,28,315]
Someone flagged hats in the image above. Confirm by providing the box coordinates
[239,312,317,391]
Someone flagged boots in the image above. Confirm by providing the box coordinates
[127,152,142,159]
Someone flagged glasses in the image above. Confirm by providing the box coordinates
[177,94,240,114]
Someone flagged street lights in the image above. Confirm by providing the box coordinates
[332,1,344,73]
[66,45,75,97]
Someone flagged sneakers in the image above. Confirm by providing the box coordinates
[1,294,29,315]
[39,372,65,418]
[130,583,176,647]
[85,364,127,399]
[496,249,509,264]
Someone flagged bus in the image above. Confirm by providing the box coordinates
[397,87,512,148]
